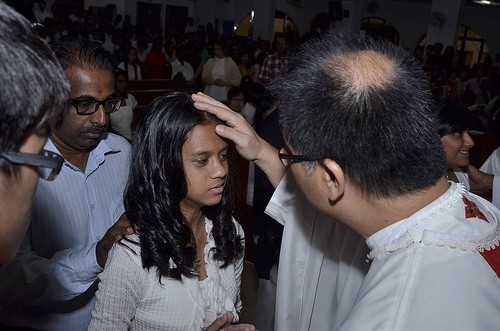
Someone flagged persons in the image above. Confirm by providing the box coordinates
[88,91,258,331]
[191,28,500,331]
[0,0,71,266]
[402,41,500,211]
[0,0,321,151]
[0,38,140,331]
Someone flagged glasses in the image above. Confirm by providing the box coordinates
[278,148,319,168]
[66,98,123,116]
[0,149,65,183]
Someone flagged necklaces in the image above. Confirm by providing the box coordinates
[191,213,205,244]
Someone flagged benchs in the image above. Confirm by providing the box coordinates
[127,78,197,130]
